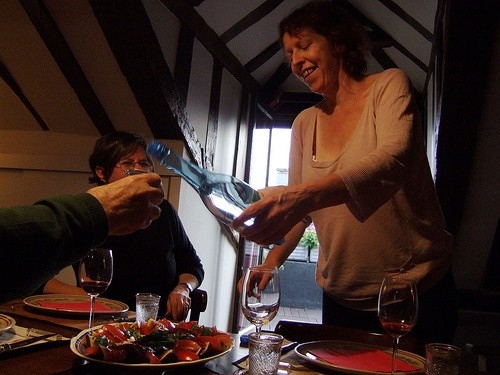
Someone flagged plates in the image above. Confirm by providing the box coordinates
[294,341,427,375]
[0,314,15,333]
[70,321,235,370]
[24,294,128,316]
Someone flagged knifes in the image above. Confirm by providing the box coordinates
[0,332,61,352]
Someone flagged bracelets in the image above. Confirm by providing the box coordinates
[180,282,193,292]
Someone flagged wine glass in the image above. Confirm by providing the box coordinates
[376,278,420,375]
[80,249,114,328]
[241,267,281,340]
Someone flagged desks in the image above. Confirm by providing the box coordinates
[0,298,321,375]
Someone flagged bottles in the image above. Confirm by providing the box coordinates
[145,142,279,250]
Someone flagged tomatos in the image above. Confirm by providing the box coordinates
[80,317,231,364]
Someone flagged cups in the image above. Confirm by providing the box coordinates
[124,168,153,176]
[425,342,462,375]
[248,332,283,375]
[136,293,160,323]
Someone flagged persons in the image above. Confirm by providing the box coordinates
[233,0,459,340]
[0,173,164,304]
[36,131,204,320]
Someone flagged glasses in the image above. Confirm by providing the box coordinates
[109,157,156,172]
